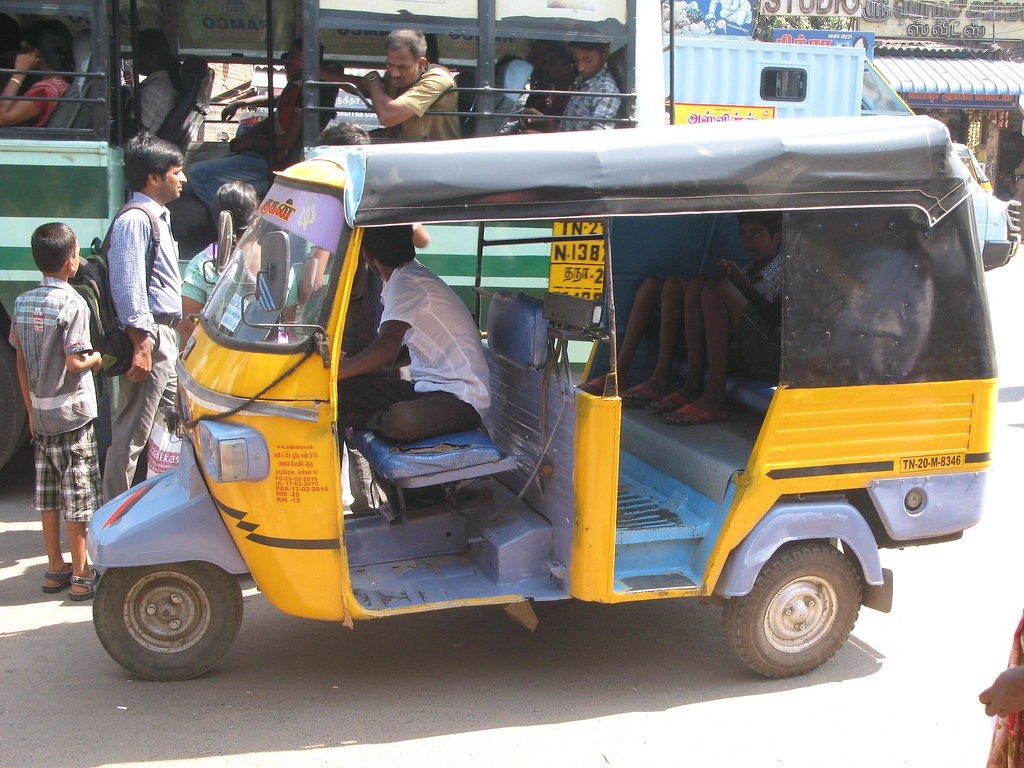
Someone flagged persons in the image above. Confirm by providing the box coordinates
[332,226,491,438]
[179,181,293,352]
[577,210,932,424]
[705,0,752,25]
[115,28,208,146]
[299,122,428,515]
[298,27,461,140]
[500,26,621,135]
[978,609,1024,768]
[0,18,75,127]
[10,222,101,600]
[187,40,324,224]
[103,133,188,505]
[853,37,868,51]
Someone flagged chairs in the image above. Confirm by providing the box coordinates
[0,30,628,232]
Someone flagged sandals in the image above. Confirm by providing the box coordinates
[68,568,102,601]
[41,563,73,594]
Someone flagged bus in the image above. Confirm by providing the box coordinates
[0,0,663,467]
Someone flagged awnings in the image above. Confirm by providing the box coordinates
[876,55,1024,95]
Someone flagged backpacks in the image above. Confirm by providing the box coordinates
[68,201,161,377]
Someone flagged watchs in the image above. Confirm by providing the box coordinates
[367,76,379,84]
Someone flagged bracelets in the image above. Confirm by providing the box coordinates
[11,78,22,87]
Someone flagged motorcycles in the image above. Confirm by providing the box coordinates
[85,114,1001,682]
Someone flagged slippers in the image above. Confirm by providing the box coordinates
[587,379,635,397]
[619,378,676,406]
[659,402,731,426]
[643,391,685,413]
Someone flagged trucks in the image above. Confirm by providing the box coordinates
[663,36,1024,272]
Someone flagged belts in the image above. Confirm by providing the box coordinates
[152,312,181,329]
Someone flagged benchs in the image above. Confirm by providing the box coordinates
[354,289,549,479]
[674,249,939,415]
